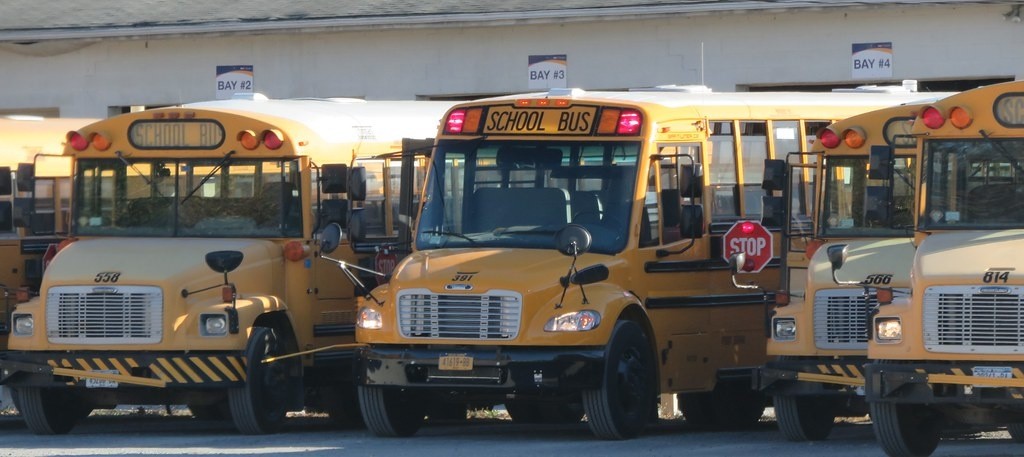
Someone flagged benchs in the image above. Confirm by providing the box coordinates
[470,186,604,231]
[967,181,1024,226]
[114,195,204,228]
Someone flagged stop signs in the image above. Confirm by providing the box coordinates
[41,244,57,274]
[723,222,775,277]
[376,252,396,285]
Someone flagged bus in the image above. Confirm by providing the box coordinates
[864,80,1024,457]
[315,73,961,441]
[769,100,967,440]
[1,96,526,433]
[0,116,293,354]
